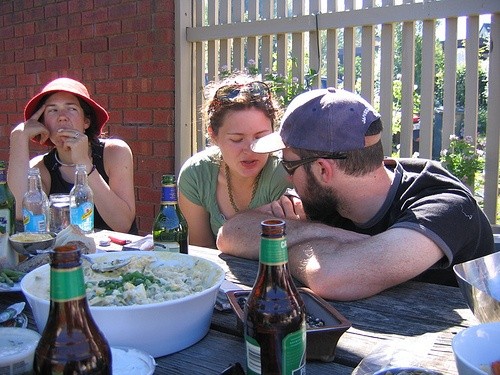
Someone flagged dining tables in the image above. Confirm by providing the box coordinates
[0,227,481,375]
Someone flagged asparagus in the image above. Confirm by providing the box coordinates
[0,269,22,287]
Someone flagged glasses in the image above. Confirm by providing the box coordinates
[212,80,272,112]
[281,153,348,177]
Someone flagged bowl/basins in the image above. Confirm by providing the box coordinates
[8,232,57,255]
[225,287,352,362]
[451,321,500,375]
[0,327,42,375]
[453,251,500,323]
[20,251,225,358]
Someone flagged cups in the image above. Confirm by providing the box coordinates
[49,193,70,235]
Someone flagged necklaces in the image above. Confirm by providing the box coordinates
[55,150,91,167]
[225,164,263,214]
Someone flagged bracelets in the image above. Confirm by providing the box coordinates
[88,165,96,177]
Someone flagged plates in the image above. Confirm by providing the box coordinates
[110,346,155,375]
[0,273,28,291]
[0,312,28,328]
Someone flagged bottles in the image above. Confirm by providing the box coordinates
[0,160,19,269]
[70,163,94,234]
[244,219,307,375]
[152,174,189,254]
[22,168,49,232]
[38,245,113,375]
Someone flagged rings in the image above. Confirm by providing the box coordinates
[74,133,79,138]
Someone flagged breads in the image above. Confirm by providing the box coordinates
[53,224,96,253]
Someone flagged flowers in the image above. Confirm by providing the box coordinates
[220,59,298,83]
[440,134,477,160]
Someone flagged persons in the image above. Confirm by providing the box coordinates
[177,74,295,250]
[6,77,137,235]
[217,87,494,302]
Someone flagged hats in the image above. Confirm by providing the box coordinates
[23,77,110,147]
[250,86,383,154]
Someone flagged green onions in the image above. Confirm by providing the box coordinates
[98,271,161,297]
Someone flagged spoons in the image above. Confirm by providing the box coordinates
[36,250,131,272]
